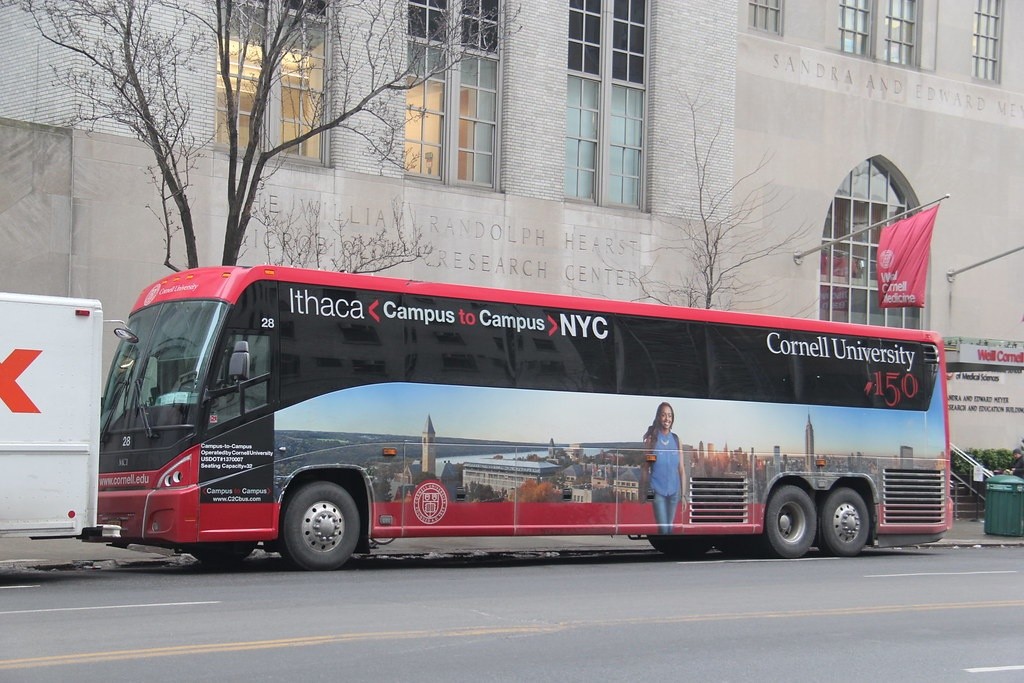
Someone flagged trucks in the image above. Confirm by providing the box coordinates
[0,291,140,541]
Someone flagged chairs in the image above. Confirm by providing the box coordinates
[228,341,250,379]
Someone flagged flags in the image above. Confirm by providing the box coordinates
[876,205,940,308]
[821,254,858,309]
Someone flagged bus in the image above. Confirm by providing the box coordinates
[77,264,955,571]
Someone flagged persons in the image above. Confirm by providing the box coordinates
[1006,448,1024,478]
[641,402,687,536]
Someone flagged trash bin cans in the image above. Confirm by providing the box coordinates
[985,473,1024,537]
